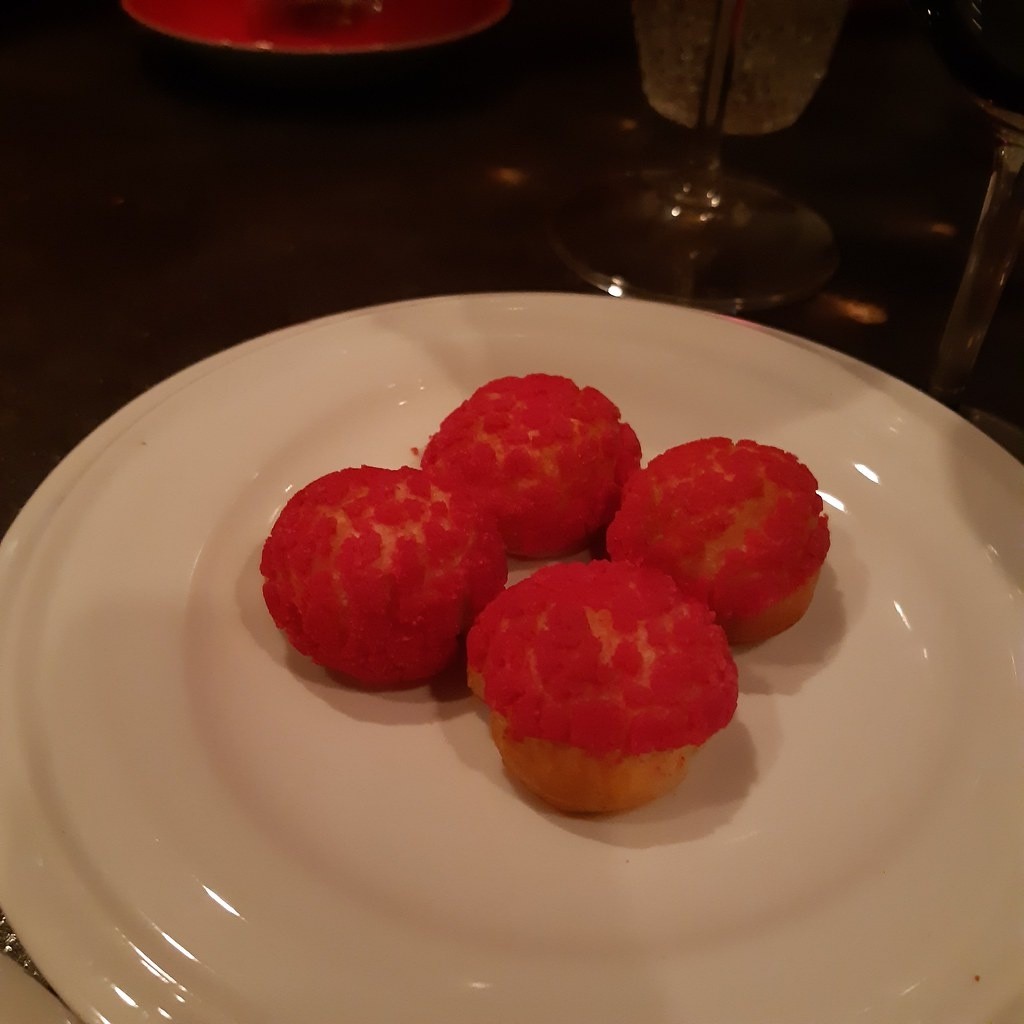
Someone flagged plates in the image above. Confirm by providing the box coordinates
[121,0,514,55]
[0,290,1024,1024]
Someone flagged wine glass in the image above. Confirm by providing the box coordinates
[888,0,1024,468]
[547,0,850,313]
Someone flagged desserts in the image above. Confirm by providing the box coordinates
[259,371,832,815]
